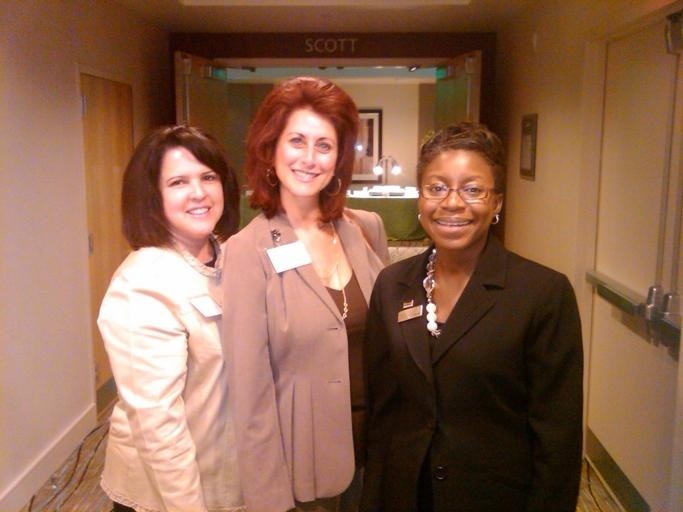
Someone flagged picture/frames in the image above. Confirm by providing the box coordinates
[350,108,382,184]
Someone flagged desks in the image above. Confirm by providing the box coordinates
[345,192,426,241]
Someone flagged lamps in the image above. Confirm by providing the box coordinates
[368,156,406,196]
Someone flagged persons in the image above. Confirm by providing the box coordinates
[363,122,583,511]
[220,78,393,512]
[96,124,238,512]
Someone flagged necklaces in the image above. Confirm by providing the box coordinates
[330,220,349,322]
[422,248,442,339]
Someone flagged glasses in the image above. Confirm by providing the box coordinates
[421,181,500,201]
